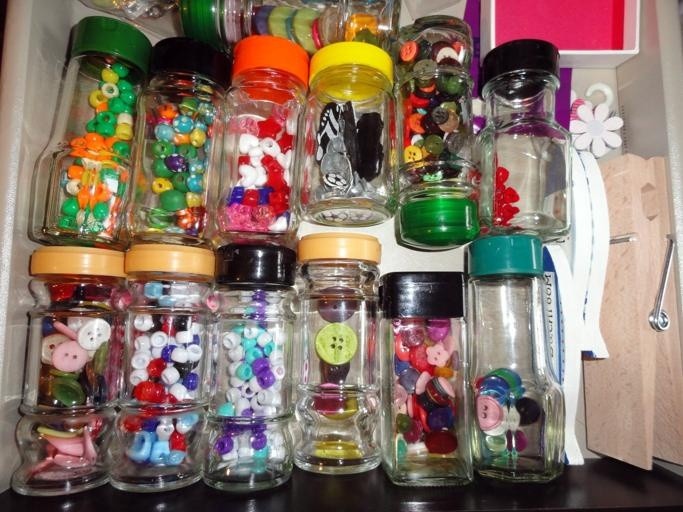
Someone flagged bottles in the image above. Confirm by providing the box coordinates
[203,34,311,250]
[110,240,214,496]
[11,232,129,499]
[464,232,568,487]
[202,242,298,497]
[71,0,402,44]
[26,16,153,250]
[378,270,475,490]
[292,233,386,477]
[120,32,234,251]
[292,13,579,250]
